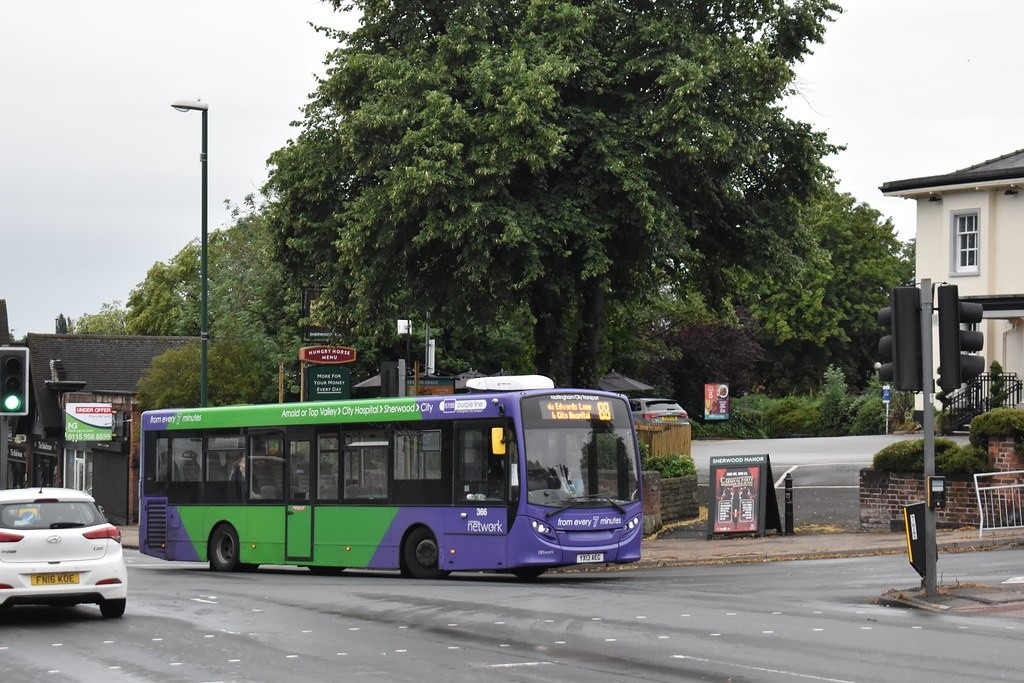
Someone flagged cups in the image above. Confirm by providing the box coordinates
[467,493,486,501]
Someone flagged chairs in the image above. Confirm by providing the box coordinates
[159,452,367,500]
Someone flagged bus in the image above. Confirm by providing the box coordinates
[138,375,641,578]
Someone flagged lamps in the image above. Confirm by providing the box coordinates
[929,197,941,201]
[1004,189,1018,195]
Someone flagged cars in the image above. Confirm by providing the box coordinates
[630,397,691,426]
[1,487,129,617]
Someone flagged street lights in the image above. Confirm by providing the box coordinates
[874,362,881,376]
[171,97,213,406]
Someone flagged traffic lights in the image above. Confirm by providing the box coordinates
[936,283,986,391]
[877,285,923,394]
[1,345,29,415]
